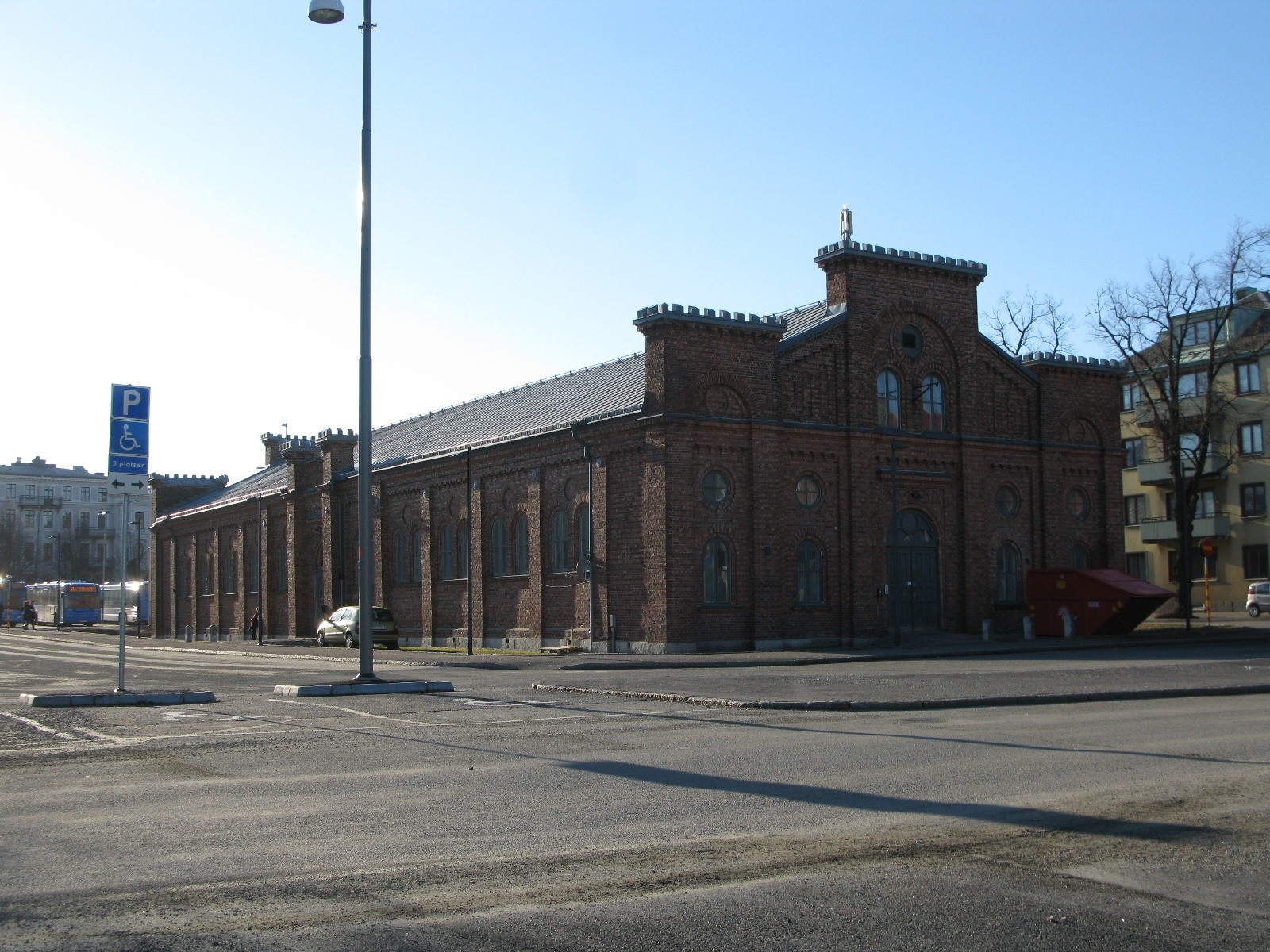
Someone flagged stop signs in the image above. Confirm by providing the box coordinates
[1197,537,1215,556]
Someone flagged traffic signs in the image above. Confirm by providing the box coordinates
[108,382,149,497]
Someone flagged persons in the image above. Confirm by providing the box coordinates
[23,601,32,630]
[30,600,38,630]
[249,618,255,641]
[253,607,260,643]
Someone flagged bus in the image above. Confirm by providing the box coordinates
[23,579,101,627]
[96,579,149,625]
[0,578,25,627]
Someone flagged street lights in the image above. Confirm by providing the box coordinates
[128,522,142,642]
[49,533,60,632]
[97,510,113,625]
[6,573,12,632]
[306,1,376,685]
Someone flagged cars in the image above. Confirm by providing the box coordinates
[317,605,399,651]
[1246,581,1270,617]
